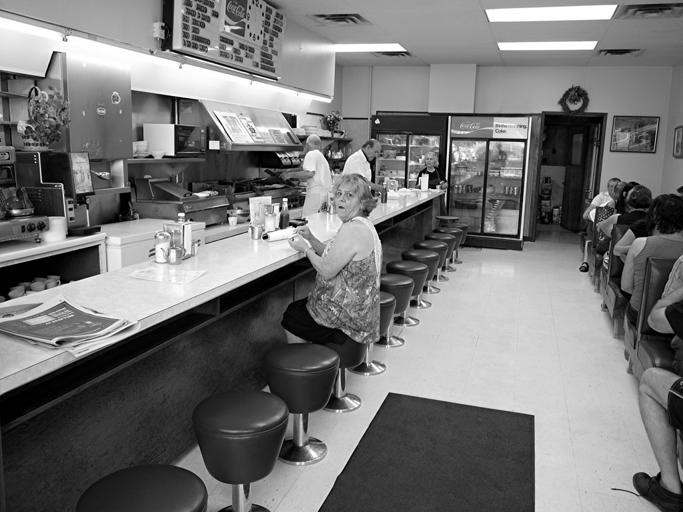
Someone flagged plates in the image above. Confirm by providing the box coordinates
[320,129,329,137]
[292,128,306,135]
[305,127,319,135]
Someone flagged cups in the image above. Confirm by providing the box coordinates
[264,213,276,231]
[20,282,31,291]
[228,217,237,226]
[388,139,392,144]
[35,278,46,281]
[163,224,183,248]
[45,279,60,289]
[30,281,45,291]
[11,286,25,294]
[8,288,24,299]
[47,276,60,280]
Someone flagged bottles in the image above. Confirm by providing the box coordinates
[504,186,509,195]
[191,239,198,256]
[155,231,171,264]
[514,187,518,196]
[381,183,387,203]
[177,213,186,225]
[454,185,458,194]
[280,198,289,229]
[459,185,462,194]
[463,185,465,194]
[509,187,513,194]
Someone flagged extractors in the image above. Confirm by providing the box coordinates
[179,100,303,151]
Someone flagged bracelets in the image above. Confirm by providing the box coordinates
[304,245,313,257]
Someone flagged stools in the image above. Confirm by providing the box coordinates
[193,390,289,512]
[385,260,429,327]
[412,240,448,294]
[435,216,460,225]
[434,228,463,272]
[400,249,440,309]
[442,224,469,264]
[347,292,396,376]
[424,233,456,282]
[262,343,340,467]
[75,464,207,512]
[374,274,414,348]
[313,330,367,414]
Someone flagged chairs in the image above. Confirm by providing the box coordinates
[623,256,648,361]
[591,208,614,288]
[628,256,682,383]
[602,224,634,320]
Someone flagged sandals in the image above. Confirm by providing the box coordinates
[579,262,588,272]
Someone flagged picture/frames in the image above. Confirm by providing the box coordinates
[611,115,660,153]
[673,126,683,158]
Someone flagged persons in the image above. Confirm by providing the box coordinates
[343,138,382,195]
[276,134,333,217]
[580,177,683,512]
[280,173,382,344]
[415,151,449,223]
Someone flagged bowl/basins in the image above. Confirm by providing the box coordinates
[151,151,165,159]
[137,141,148,153]
[133,142,137,154]
[229,209,250,223]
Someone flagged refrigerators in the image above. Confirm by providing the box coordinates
[371,114,445,217]
[444,114,542,249]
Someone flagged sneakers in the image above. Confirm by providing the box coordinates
[632,471,682,511]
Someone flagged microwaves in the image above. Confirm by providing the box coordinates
[142,122,208,157]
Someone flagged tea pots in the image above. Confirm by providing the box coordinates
[161,247,187,265]
[419,174,429,192]
[248,223,264,240]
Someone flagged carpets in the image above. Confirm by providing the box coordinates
[318,391,535,511]
